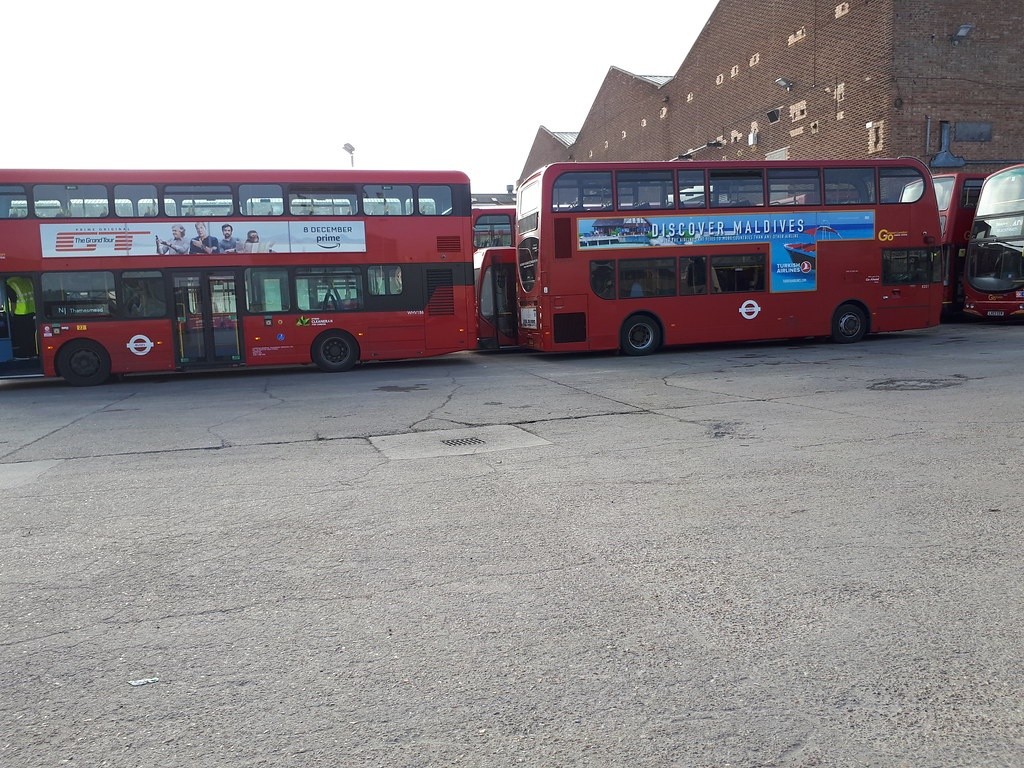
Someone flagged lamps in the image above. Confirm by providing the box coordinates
[774,76,792,94]
[952,25,971,42]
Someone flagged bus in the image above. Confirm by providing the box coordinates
[962,166,1024,321]
[898,172,1024,311]
[507,139,947,359]
[431,203,751,353]
[754,188,865,206]
[1,169,481,387]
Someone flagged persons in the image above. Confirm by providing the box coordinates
[155,223,189,256]
[189,221,218,254]
[683,257,722,293]
[218,223,240,253]
[6,275,36,358]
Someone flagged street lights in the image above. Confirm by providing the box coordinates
[343,143,356,168]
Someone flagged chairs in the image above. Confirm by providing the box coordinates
[570,195,919,211]
[9,204,450,218]
[481,230,504,246]
